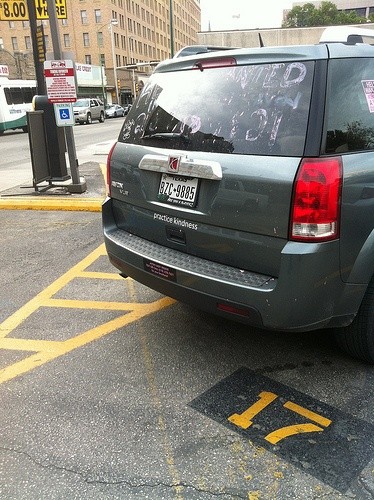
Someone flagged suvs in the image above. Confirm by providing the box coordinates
[72,98,104,125]
[101,25,374,365]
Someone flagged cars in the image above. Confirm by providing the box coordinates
[104,104,125,118]
[120,104,132,116]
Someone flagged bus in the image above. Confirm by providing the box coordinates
[0,77,40,136]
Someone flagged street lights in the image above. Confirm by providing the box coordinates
[108,21,120,104]
